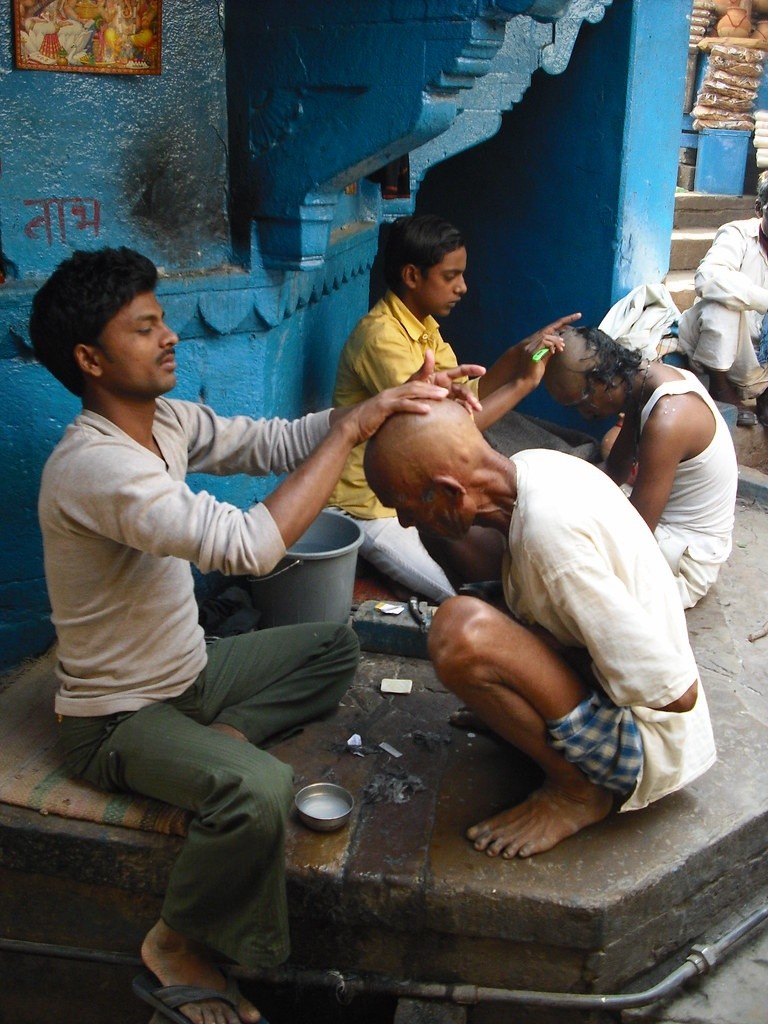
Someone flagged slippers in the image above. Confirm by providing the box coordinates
[132,971,269,1024]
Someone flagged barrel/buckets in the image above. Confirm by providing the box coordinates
[249,509,365,630]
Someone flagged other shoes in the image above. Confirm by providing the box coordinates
[756,409,768,427]
[718,397,758,425]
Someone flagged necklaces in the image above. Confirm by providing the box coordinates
[629,358,651,476]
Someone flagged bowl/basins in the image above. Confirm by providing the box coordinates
[294,782,355,831]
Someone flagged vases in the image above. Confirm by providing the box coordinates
[751,21,768,40]
[716,7,751,38]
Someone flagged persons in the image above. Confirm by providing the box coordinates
[363,397,717,860]
[545,324,738,612]
[29,247,487,1024]
[327,213,584,604]
[678,169,768,427]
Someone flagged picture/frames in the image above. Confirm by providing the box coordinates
[12,0,162,75]
